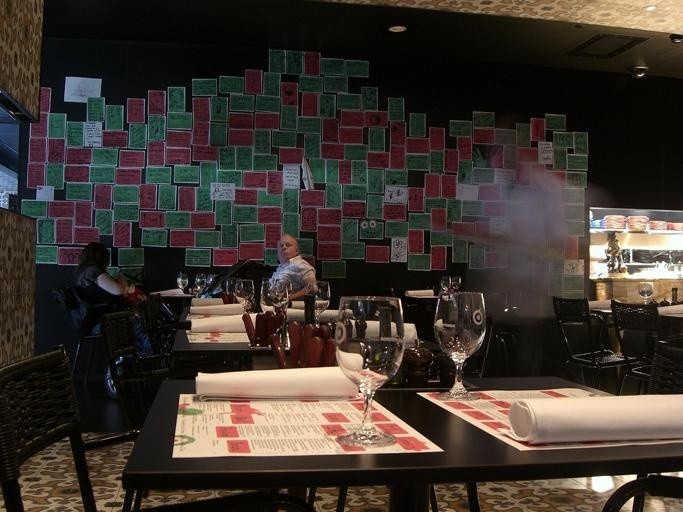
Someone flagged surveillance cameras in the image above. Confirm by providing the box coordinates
[670,34,683,43]
[630,66,649,79]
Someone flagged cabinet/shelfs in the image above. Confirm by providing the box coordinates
[589,207,683,309]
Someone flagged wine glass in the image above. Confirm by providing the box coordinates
[333,296,406,449]
[638,281,654,304]
[433,277,487,401]
[175,273,330,347]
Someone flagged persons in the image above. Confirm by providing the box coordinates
[73,242,152,353]
[261,233,319,301]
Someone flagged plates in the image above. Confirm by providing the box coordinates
[590,214,683,231]
